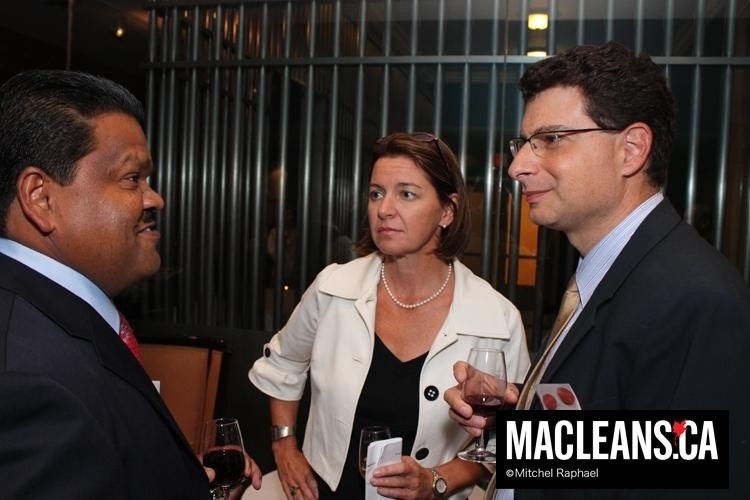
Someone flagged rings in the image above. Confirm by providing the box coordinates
[289,486,300,496]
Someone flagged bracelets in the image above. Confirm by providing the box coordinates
[265,423,296,443]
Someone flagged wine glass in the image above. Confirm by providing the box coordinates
[203,418,244,500]
[457,348,508,463]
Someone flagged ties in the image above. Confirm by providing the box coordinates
[484,273,580,499]
[119,311,142,367]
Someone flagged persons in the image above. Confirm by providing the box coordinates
[443,43,750,500]
[0,69,262,500]
[248,133,531,500]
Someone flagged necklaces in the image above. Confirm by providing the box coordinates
[380,258,453,309]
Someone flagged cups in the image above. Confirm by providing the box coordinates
[358,425,391,480]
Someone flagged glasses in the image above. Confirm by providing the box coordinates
[508,128,620,159]
[375,132,449,173]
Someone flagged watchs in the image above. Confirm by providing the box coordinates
[429,467,447,500]
